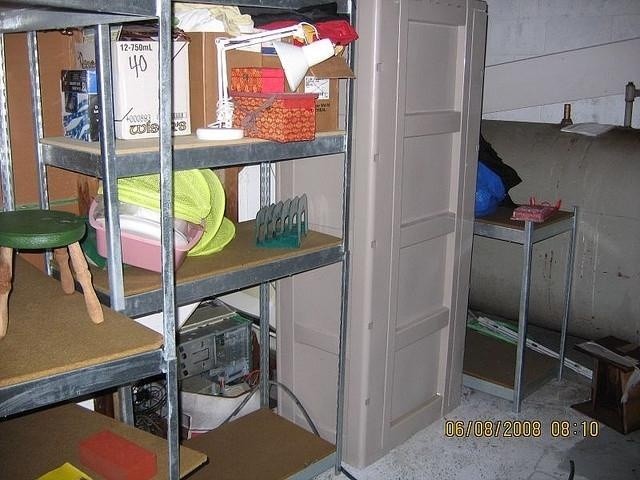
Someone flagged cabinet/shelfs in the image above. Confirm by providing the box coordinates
[463,204,579,415]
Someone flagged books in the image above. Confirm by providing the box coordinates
[560,121,616,137]
[573,334,639,373]
